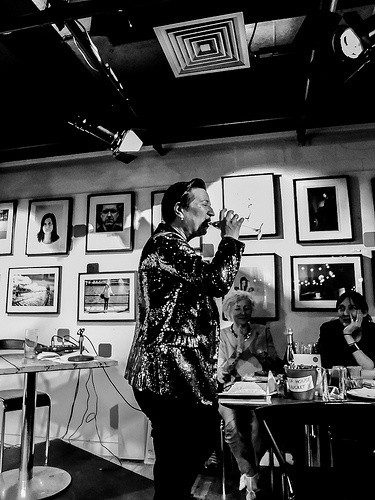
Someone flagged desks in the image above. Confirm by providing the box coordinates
[216,386,375,500]
[0,349,118,500]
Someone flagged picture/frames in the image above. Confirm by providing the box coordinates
[371,250,375,307]
[77,270,137,323]
[221,174,281,238]
[370,176,375,213]
[0,199,18,257]
[290,254,366,313]
[5,266,63,314]
[292,174,355,243]
[85,191,135,253]
[150,190,203,252]
[25,197,74,256]
[222,253,281,323]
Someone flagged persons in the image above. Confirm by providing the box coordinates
[37,213,59,244]
[217,294,283,500]
[124,178,245,500]
[101,284,114,310]
[97,203,123,232]
[316,290,375,370]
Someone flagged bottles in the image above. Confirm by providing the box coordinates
[281,328,296,373]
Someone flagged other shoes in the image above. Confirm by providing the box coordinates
[239,473,260,500]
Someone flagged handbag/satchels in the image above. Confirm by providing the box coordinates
[101,294,104,298]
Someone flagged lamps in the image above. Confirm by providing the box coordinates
[67,112,144,165]
[330,9,375,85]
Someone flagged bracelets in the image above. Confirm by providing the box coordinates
[343,334,351,335]
[348,343,359,353]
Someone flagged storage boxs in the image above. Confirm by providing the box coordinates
[292,354,324,396]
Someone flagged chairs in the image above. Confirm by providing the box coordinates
[0,338,53,475]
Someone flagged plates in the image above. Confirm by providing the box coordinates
[346,386,375,399]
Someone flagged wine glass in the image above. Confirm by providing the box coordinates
[207,210,264,240]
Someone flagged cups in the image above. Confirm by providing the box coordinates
[319,365,365,403]
[24,327,40,359]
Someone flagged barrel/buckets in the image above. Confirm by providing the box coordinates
[285,367,320,400]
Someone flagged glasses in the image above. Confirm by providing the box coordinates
[103,208,117,213]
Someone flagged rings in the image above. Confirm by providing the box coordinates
[356,320,358,322]
